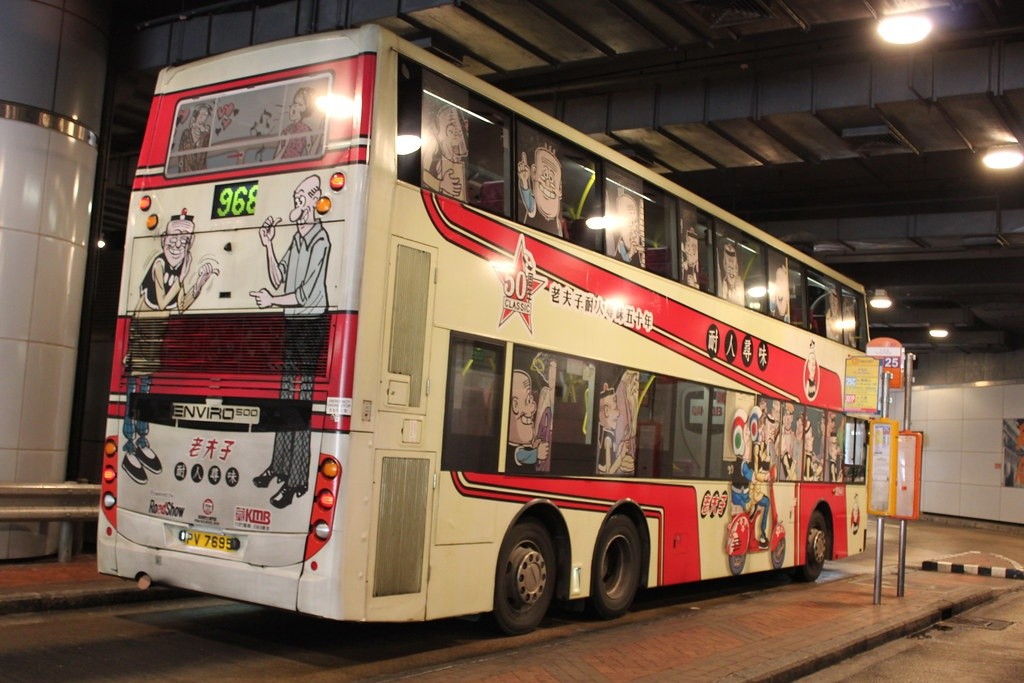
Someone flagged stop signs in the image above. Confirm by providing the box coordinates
[865,338,903,366]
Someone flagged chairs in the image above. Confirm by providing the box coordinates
[441,432,720,481]
[463,178,828,340]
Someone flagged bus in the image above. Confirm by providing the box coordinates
[94,21,874,636]
